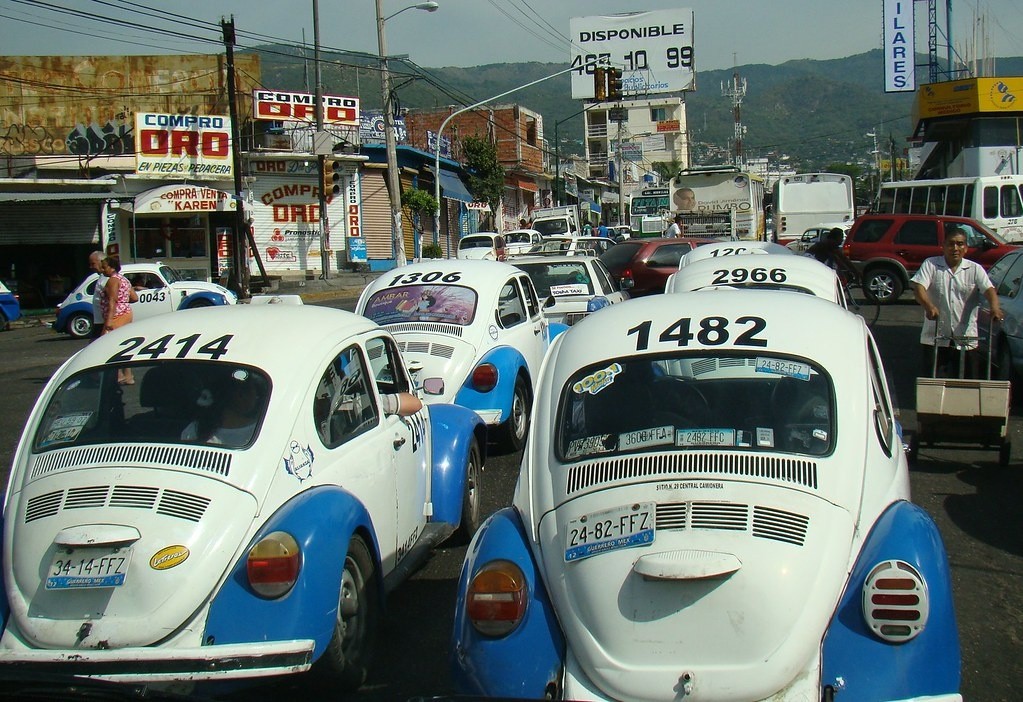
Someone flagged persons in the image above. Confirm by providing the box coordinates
[665,216,684,239]
[131,274,148,289]
[806,228,864,279]
[597,222,610,252]
[100,257,139,384]
[674,188,696,210]
[77,251,110,387]
[581,219,592,236]
[520,219,531,229]
[909,227,1005,379]
[335,357,422,416]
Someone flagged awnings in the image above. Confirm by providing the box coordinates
[600,190,630,204]
[576,193,600,214]
[0,192,136,202]
[120,184,253,214]
[505,178,537,205]
[427,165,472,204]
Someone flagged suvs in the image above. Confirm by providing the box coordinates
[844,210,1023,304]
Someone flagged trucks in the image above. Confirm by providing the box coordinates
[772,172,853,247]
[531,203,580,256]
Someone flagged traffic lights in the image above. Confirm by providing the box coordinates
[608,67,622,101]
[323,159,339,197]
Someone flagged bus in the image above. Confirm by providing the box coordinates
[670,165,766,240]
[871,174,1023,242]
[629,188,669,238]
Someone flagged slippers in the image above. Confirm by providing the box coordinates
[118,377,135,386]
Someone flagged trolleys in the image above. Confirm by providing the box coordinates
[909,313,1011,467]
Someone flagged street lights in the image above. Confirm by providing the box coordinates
[618,131,651,225]
[376,2,438,267]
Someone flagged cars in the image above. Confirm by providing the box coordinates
[663,243,847,310]
[606,225,630,240]
[971,246,1023,414]
[354,237,717,452]
[0,296,487,702]
[0,281,21,331]
[457,229,543,262]
[451,286,961,702]
[53,260,242,339]
